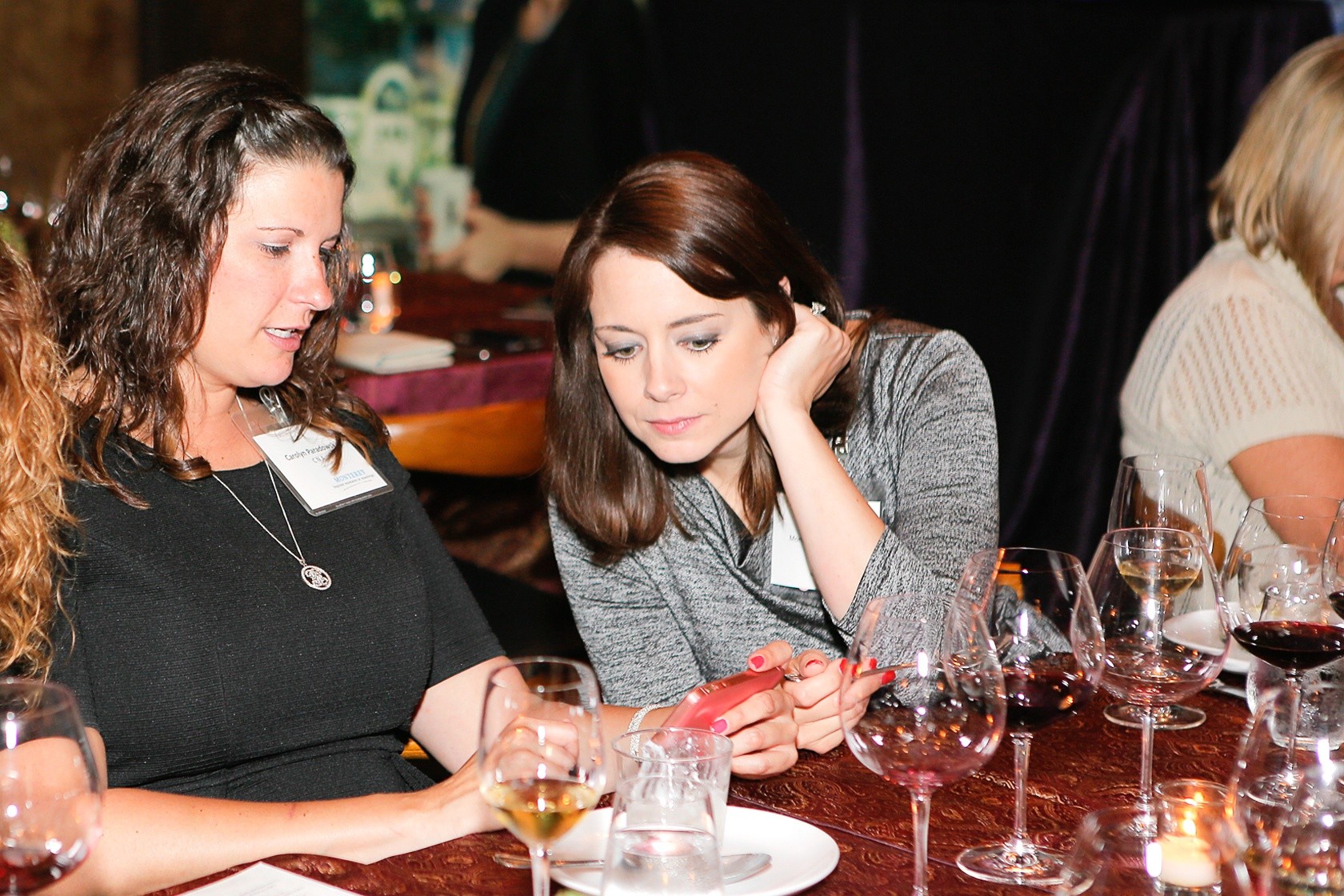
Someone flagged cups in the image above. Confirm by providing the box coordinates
[343,240,393,319]
[420,167,471,250]
[1237,543,1344,750]
[606,727,735,896]
[1232,689,1344,896]
[1053,804,1249,896]
[0,678,108,896]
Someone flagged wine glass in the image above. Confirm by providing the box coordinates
[1069,528,1231,843]
[1218,496,1344,808]
[840,592,1006,896]
[478,657,605,896]
[1102,454,1214,729]
[940,547,1105,885]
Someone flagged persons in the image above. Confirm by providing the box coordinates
[0,60,798,896]
[1121,33,1344,619]
[539,154,1001,760]
[411,0,646,304]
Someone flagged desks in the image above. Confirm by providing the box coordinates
[347,306,556,481]
[144,634,1343,896]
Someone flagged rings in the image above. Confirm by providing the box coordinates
[810,302,827,315]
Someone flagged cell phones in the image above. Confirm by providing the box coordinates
[650,667,787,748]
[450,328,542,361]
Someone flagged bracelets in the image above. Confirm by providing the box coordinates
[627,702,675,755]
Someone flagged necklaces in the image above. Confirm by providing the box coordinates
[120,381,333,591]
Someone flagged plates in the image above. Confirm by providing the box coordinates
[550,806,840,896]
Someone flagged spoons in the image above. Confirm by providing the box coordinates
[492,851,771,884]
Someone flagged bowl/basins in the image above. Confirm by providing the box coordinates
[1157,606,1248,690]
